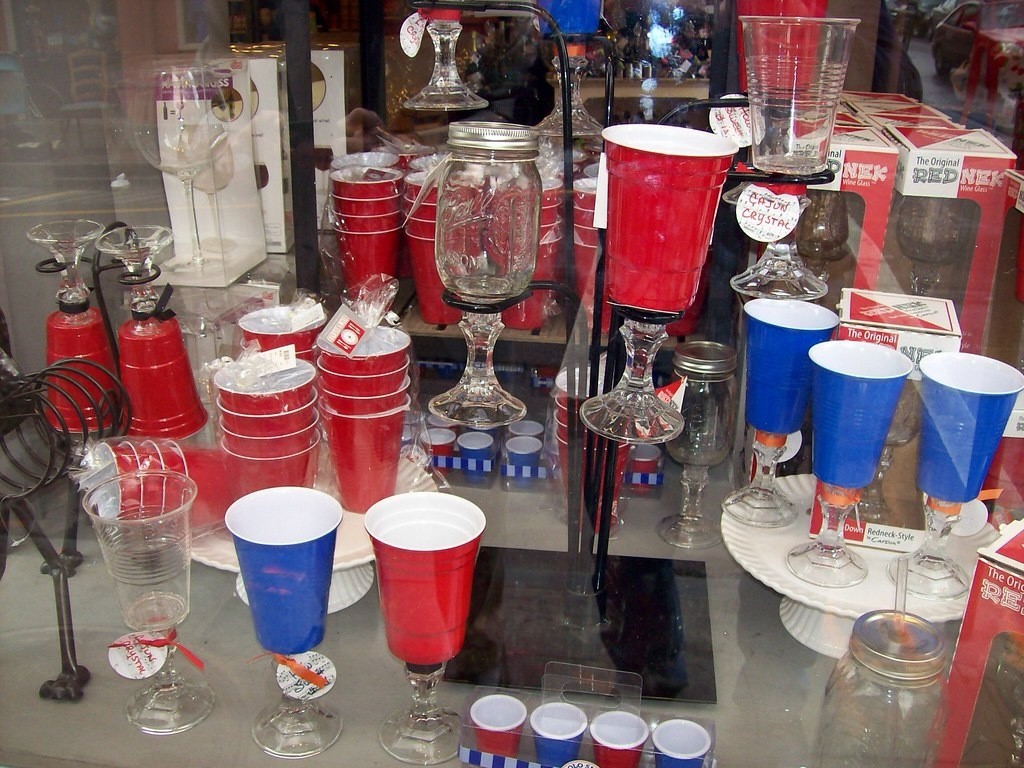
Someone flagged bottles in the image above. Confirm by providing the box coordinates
[814,611,947,768]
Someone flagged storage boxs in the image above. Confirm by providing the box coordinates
[746,90,1024,768]
[155,41,362,360]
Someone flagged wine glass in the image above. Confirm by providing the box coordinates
[657,288,1024,602]
[82,472,487,765]
[578,123,739,448]
[427,118,540,431]
[723,1,861,301]
[401,0,490,109]
[179,124,236,255]
[534,0,605,135]
[134,67,233,278]
[25,217,208,444]
[795,188,972,305]
[986,632,1024,768]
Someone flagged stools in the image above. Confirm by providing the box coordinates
[960,27,1024,133]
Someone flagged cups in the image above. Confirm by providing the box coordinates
[401,368,661,532]
[325,141,712,335]
[470,695,712,768]
[211,304,411,514]
